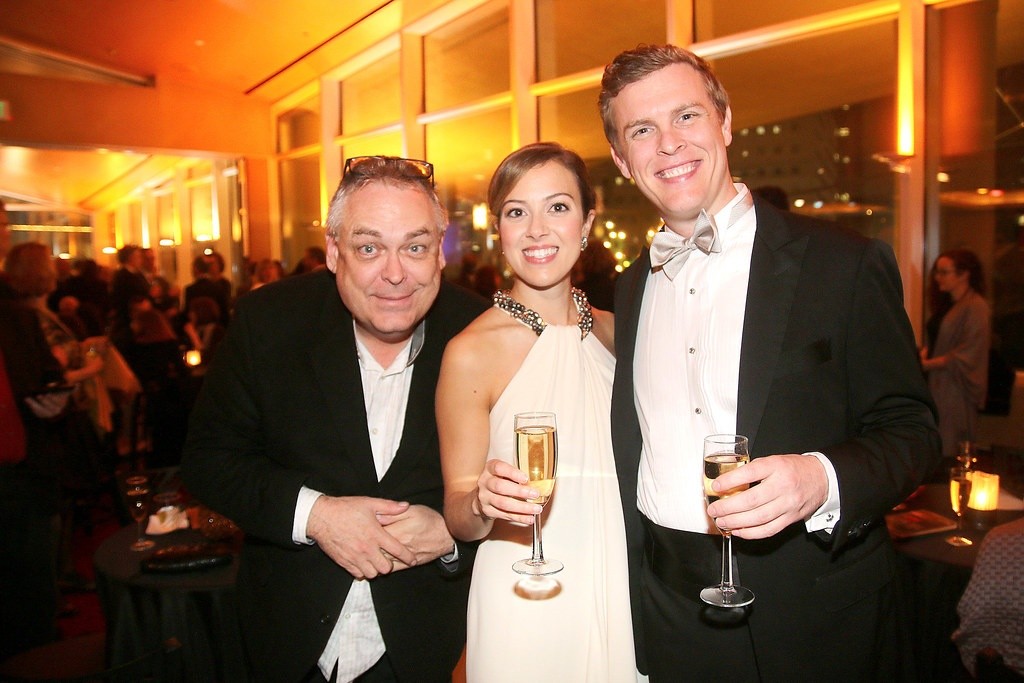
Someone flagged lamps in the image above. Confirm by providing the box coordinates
[952,470,1001,523]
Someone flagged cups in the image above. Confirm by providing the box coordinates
[125,476,148,489]
[151,467,186,510]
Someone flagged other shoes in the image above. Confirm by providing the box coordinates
[114,497,135,526]
[49,573,90,594]
[55,600,82,616]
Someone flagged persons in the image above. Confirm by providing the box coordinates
[919,249,992,459]
[601,46,941,683]
[0,201,232,641]
[446,254,502,301]
[980,218,1024,417]
[578,243,617,312]
[188,156,492,683]
[304,246,327,271]
[239,256,283,292]
[434,144,649,682]
[753,185,791,211]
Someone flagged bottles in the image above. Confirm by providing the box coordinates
[947,440,986,472]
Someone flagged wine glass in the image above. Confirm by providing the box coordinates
[699,433,757,610]
[946,467,974,547]
[511,411,565,578]
[124,489,155,551]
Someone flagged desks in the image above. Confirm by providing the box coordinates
[885,451,1024,683]
[92,511,267,683]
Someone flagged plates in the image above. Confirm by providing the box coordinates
[886,509,958,539]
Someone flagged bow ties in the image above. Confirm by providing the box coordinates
[649,189,755,282]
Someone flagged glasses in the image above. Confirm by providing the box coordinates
[343,155,434,189]
[935,266,958,276]
